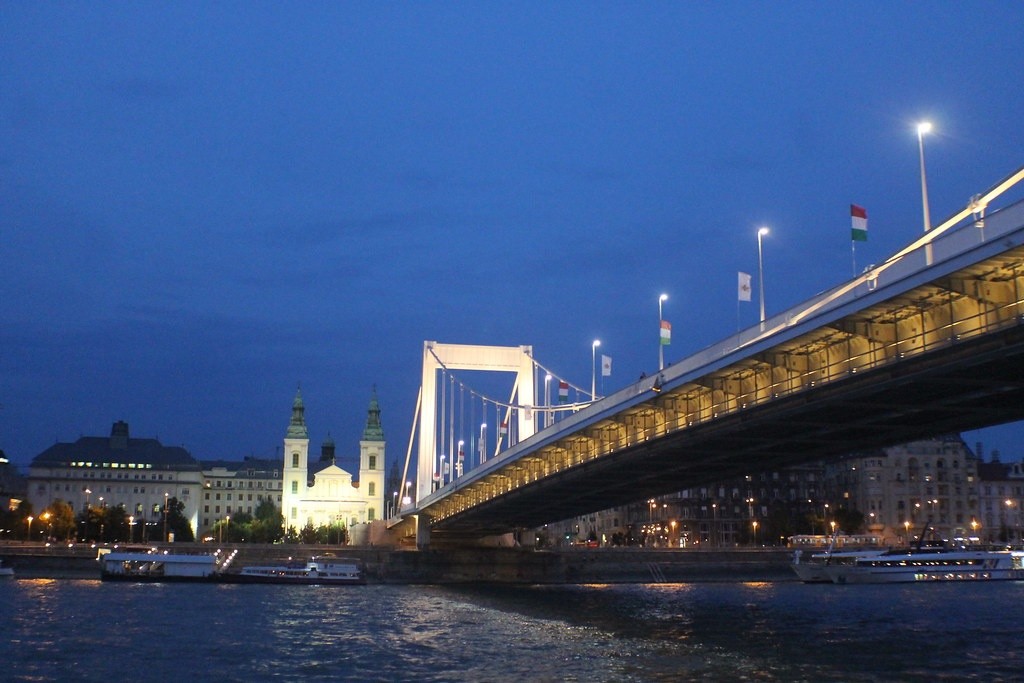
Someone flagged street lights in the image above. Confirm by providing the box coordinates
[84,488,92,542]
[28,512,52,541]
[746,496,753,522]
[163,493,169,541]
[457,440,464,477]
[393,482,412,517]
[647,497,654,548]
[757,227,770,331]
[753,522,757,547]
[659,295,668,370]
[592,340,600,399]
[226,516,230,543]
[918,120,933,267]
[712,503,717,547]
[480,423,486,464]
[544,375,552,428]
[129,516,134,544]
[672,521,676,543]
[440,455,445,488]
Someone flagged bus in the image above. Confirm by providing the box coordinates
[787,534,884,549]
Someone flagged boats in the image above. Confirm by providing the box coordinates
[790,515,1024,585]
[100,548,219,582]
[238,556,368,585]
